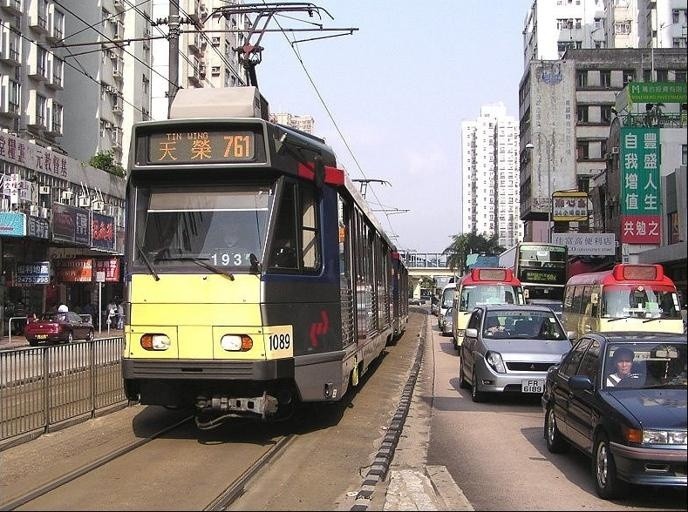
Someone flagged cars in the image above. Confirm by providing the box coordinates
[437,307,453,337]
[455,303,573,405]
[22,303,95,348]
[538,329,687,500]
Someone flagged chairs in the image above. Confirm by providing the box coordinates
[486,318,535,335]
[574,350,642,386]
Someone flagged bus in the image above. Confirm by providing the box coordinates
[430,275,455,317]
[496,240,570,318]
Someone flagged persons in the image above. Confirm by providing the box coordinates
[606,348,635,387]
[13,300,125,336]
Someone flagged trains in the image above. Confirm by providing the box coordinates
[116,0,409,434]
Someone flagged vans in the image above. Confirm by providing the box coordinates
[451,265,528,357]
[559,261,683,348]
[437,282,456,330]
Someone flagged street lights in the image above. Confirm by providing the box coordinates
[524,130,553,243]
